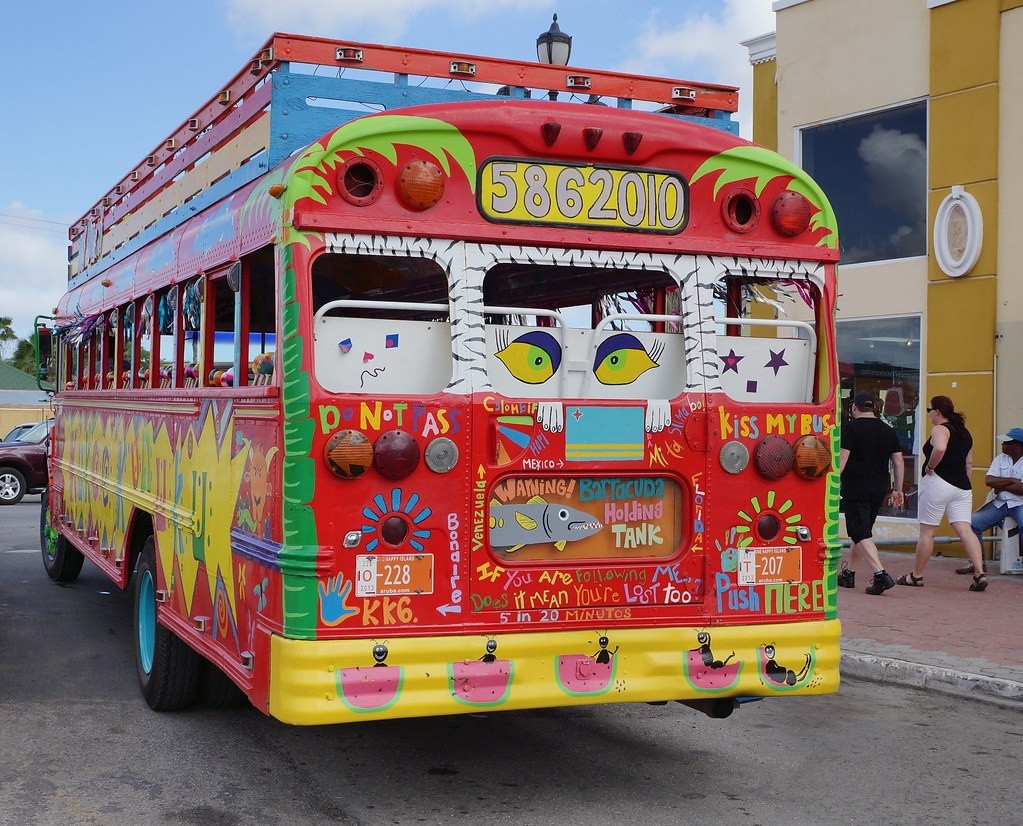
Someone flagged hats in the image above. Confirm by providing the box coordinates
[995,428,1023,442]
[849,392,874,407]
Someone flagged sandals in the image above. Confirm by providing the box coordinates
[894,571,924,586]
[967,573,988,591]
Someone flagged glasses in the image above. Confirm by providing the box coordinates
[926,408,934,413]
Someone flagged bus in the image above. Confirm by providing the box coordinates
[34,32,842,727]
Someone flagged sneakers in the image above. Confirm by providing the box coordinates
[865,574,894,595]
[954,560,987,576]
[837,561,856,588]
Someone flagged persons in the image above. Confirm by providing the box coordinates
[837,390,904,595]
[954,427,1023,575]
[896,395,989,591]
[40,347,48,368]
[83,336,130,376]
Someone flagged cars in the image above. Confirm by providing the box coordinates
[0,418,55,505]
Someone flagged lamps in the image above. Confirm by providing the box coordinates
[868,341,875,349]
[904,340,913,350]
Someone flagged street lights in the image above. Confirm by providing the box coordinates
[537,13,573,102]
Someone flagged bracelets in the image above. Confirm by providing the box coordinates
[928,466,933,470]
[894,488,903,492]
